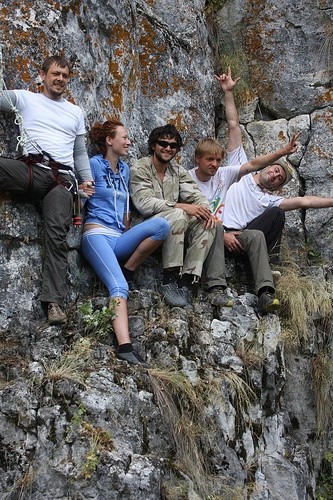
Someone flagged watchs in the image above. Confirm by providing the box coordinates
[82,181,94,186]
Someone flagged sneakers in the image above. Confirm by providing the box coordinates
[47,302,67,324]
[258,291,280,312]
[159,281,186,307]
[178,274,198,294]
[197,286,234,307]
[270,263,281,282]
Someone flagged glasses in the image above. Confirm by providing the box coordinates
[285,161,293,174]
[156,139,178,149]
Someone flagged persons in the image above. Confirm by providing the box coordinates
[71,120,170,365]
[128,123,216,308]
[190,130,302,308]
[213,65,333,316]
[0,55,95,326]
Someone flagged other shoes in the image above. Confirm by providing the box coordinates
[122,269,140,294]
[116,350,150,369]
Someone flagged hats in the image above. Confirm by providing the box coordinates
[265,159,293,192]
